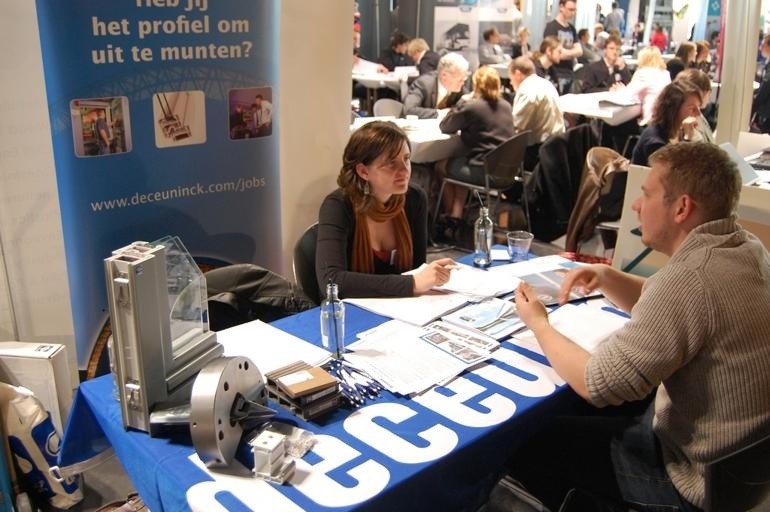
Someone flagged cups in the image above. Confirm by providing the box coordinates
[506,231,535,258]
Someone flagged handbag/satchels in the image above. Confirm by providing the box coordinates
[0,384,85,510]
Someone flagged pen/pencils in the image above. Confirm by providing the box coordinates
[444,265,463,271]
[321,358,387,409]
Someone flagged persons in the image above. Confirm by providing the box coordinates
[437,64,518,238]
[94,108,118,155]
[514,141,770,462]
[628,82,703,164]
[250,103,257,126]
[255,94,272,132]
[508,56,566,142]
[228,105,245,127]
[314,120,454,296]
[350,2,770,145]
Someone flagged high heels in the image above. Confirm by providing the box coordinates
[437,217,462,238]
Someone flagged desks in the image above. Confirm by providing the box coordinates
[79,238,638,512]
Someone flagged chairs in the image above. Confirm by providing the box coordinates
[170,260,318,336]
[555,432,770,511]
[293,219,325,307]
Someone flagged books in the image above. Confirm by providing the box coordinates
[393,320,502,402]
[717,142,761,187]
[339,259,525,327]
[486,253,606,306]
[340,321,493,399]
[275,365,339,398]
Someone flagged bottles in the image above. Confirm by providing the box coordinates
[319,283,345,360]
[474,207,492,265]
